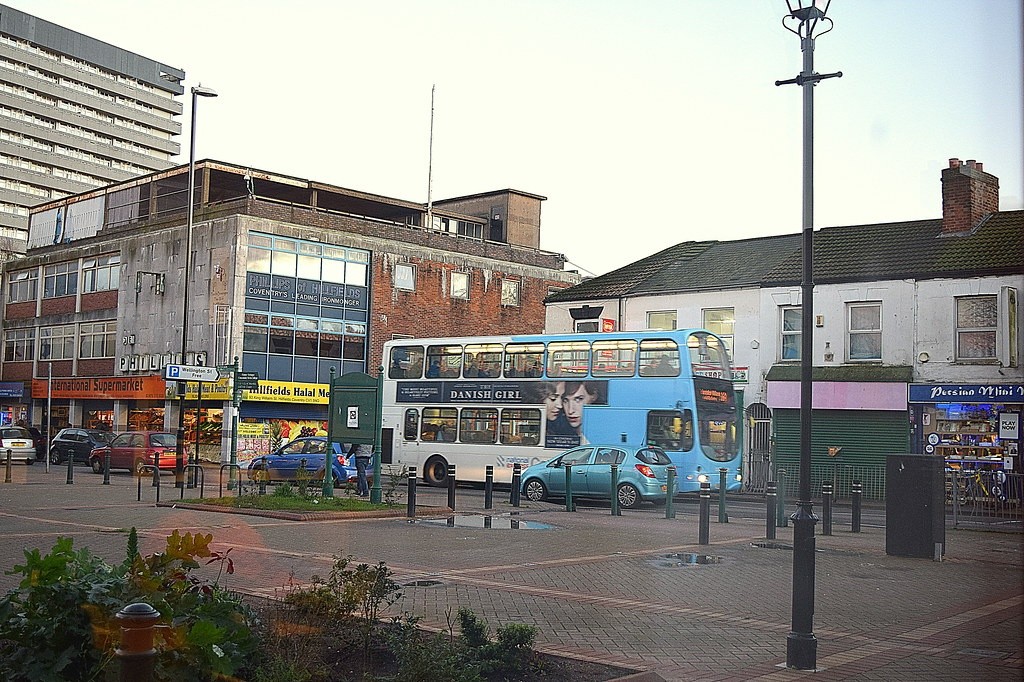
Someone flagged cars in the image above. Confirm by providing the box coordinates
[0,425,38,466]
[88,430,189,476]
[519,443,679,510]
[247,435,374,489]
[50,426,129,468]
[25,426,47,457]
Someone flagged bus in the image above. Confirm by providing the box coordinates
[379,328,745,506]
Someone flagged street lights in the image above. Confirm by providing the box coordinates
[173,85,219,492]
[775,0,843,675]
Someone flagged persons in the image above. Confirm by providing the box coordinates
[393,354,677,378]
[518,381,569,446]
[347,443,375,496]
[561,381,606,446]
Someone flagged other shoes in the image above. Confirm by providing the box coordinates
[353,492,360,496]
[360,492,369,498]
